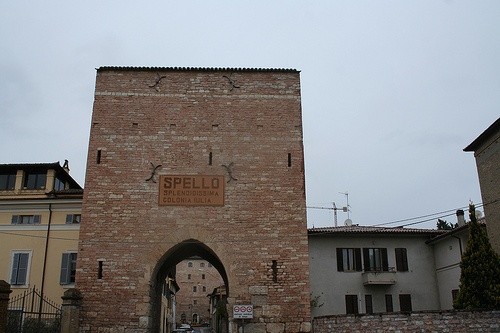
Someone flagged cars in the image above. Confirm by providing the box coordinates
[177,323,194,333]
